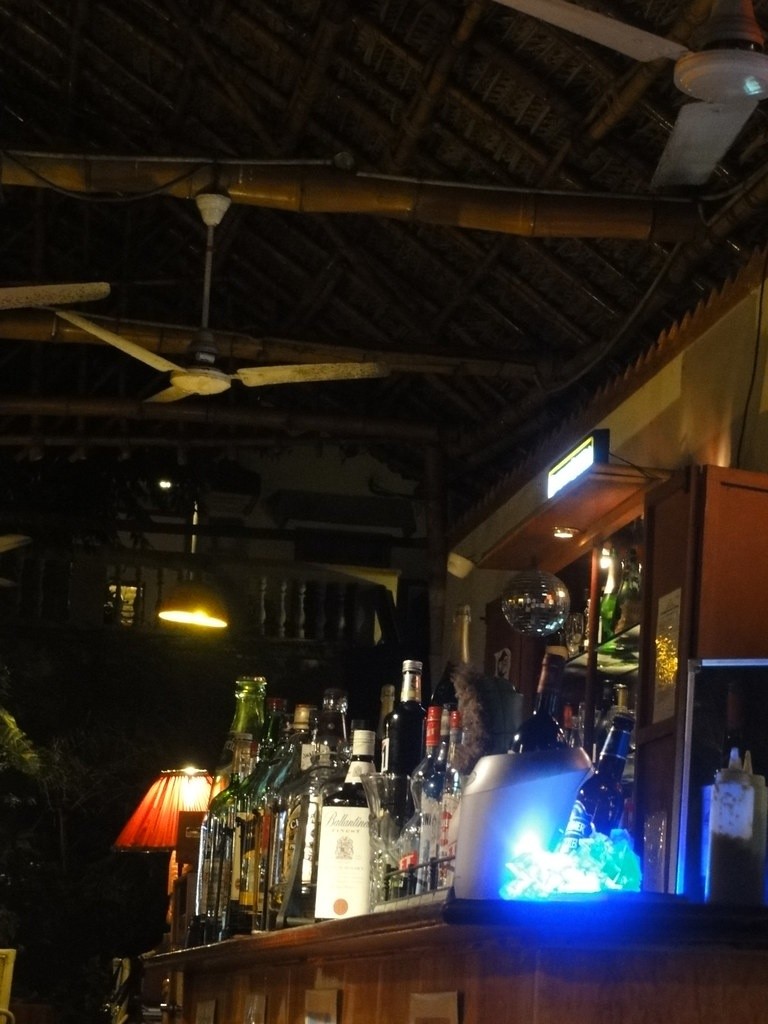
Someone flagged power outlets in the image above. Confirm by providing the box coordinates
[91,831,111,859]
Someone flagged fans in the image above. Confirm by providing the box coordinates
[493,0,768,194]
[0,193,392,403]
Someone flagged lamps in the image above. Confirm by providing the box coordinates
[551,527,580,539]
[542,428,610,500]
[158,579,229,631]
[103,579,144,638]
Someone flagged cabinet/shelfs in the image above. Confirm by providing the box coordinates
[137,888,768,1024]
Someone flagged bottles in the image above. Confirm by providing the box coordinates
[194,659,466,945]
[704,747,767,905]
[508,547,643,893]
